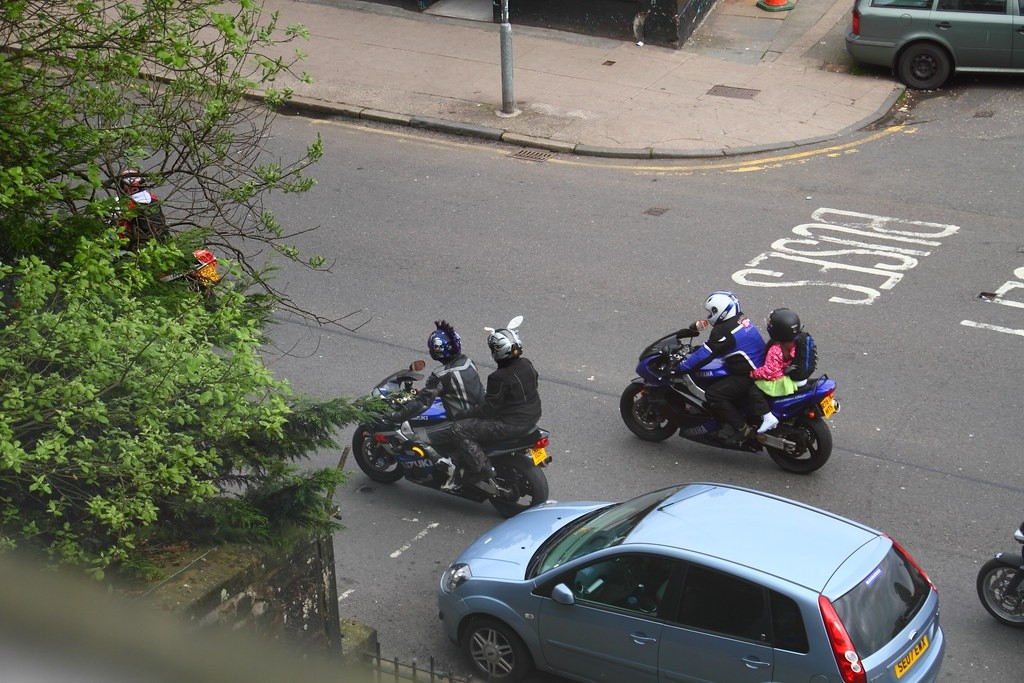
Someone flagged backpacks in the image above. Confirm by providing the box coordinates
[784,332,817,381]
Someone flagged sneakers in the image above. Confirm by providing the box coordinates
[757,412,778,433]
[726,425,755,446]
[440,457,465,492]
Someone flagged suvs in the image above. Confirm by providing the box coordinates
[434,481,947,683]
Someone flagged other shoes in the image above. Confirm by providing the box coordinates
[464,464,496,484]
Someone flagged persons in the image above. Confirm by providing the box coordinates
[382,319,485,491]
[449,328,542,484]
[749,308,809,433]
[664,290,766,446]
[106,170,171,264]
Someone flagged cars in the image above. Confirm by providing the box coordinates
[845,0,1024,89]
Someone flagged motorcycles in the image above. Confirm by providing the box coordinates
[975,519,1024,628]
[153,249,218,311]
[351,361,552,518]
[618,324,838,474]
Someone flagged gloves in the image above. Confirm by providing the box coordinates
[382,413,393,420]
[664,365,680,378]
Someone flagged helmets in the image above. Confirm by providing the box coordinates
[427,320,461,360]
[704,290,741,327]
[484,315,524,363]
[117,170,146,196]
[767,308,800,341]
